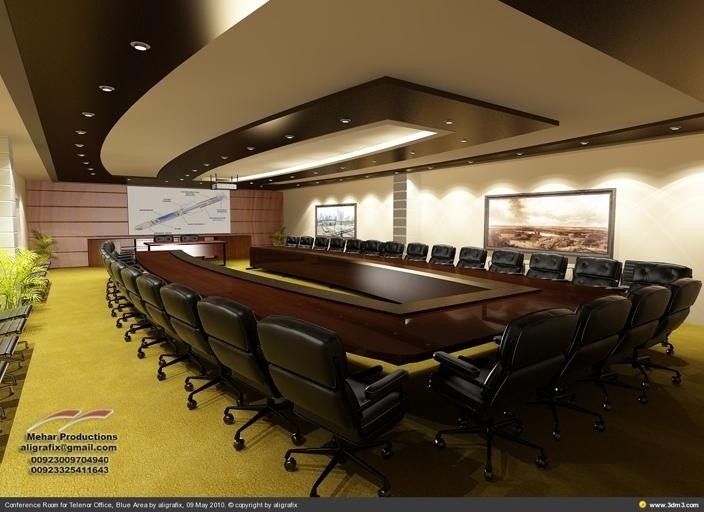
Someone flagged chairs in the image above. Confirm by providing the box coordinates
[645,278,702,355]
[623,261,692,295]
[427,309,580,481]
[284,236,526,289]
[574,255,621,288]
[551,296,632,438]
[527,253,568,281]
[607,284,681,408]
[0,305,31,435]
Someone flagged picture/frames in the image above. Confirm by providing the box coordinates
[483,188,617,262]
[314,203,356,239]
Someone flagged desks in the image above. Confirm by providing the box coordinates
[88,235,252,269]
[129,241,630,405]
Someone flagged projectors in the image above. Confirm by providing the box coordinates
[212,183,237,189]
